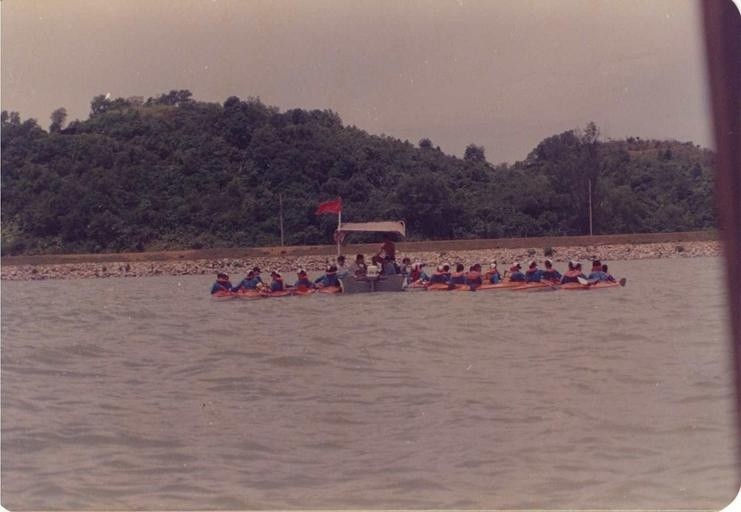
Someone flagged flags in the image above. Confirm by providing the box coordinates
[316,200,342,215]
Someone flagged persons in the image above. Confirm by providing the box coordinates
[211,234,615,293]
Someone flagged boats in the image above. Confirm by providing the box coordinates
[212,282,342,301]
[333,194,410,294]
[403,277,621,291]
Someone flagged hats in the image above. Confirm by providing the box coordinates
[411,262,426,273]
[510,262,521,270]
[568,260,583,269]
[545,259,554,266]
[528,261,539,268]
[437,263,451,272]
[489,263,497,271]
[217,265,337,280]
